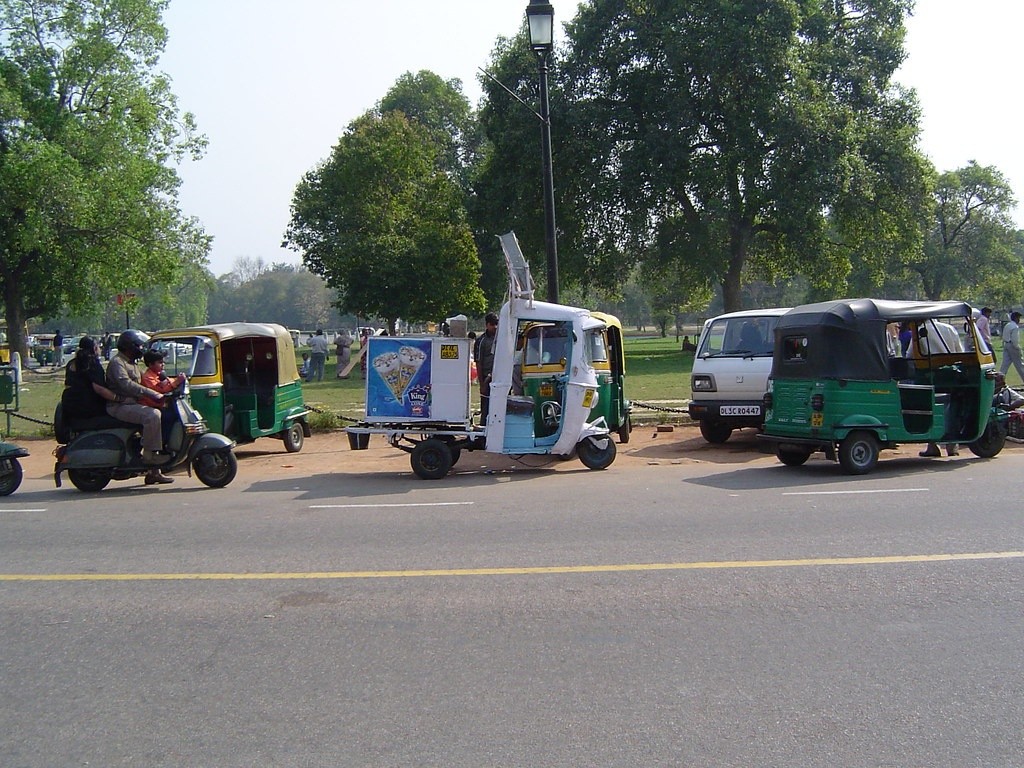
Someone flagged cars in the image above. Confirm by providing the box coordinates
[63,339,71,346]
[65,337,82,354]
[354,327,375,336]
[161,341,192,354]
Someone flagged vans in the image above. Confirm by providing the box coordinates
[688,308,796,443]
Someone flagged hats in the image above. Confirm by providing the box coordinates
[485,312,499,321]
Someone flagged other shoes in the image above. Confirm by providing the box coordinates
[919,443,941,457]
[946,443,959,456]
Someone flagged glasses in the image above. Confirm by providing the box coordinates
[487,321,498,326]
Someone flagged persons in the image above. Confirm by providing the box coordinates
[305,329,329,382]
[474,312,499,426]
[898,322,912,357]
[999,309,1010,348]
[906,319,964,456]
[886,322,898,357]
[141,349,186,457]
[62,336,137,420]
[106,329,175,484]
[52,330,63,367]
[298,353,316,378]
[976,307,997,365]
[683,336,694,351]
[103,332,112,361]
[999,312,1024,382]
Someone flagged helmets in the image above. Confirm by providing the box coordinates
[116,329,151,359]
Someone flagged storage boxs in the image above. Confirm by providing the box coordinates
[449,319,468,338]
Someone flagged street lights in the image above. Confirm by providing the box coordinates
[525,0,560,304]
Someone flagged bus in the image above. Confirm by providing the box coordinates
[0,319,29,366]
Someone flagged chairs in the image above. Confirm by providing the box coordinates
[892,358,951,404]
[736,323,764,351]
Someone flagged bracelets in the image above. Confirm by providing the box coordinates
[113,394,116,401]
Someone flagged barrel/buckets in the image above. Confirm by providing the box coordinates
[347,432,370,450]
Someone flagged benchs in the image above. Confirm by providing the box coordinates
[507,394,536,416]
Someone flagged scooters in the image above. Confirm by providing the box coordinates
[147,322,313,452]
[0,442,30,496]
[33,335,54,366]
[755,298,1006,474]
[52,370,237,493]
[345,299,616,479]
[521,312,633,443]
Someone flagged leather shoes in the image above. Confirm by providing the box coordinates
[145,473,175,485]
[142,453,170,465]
